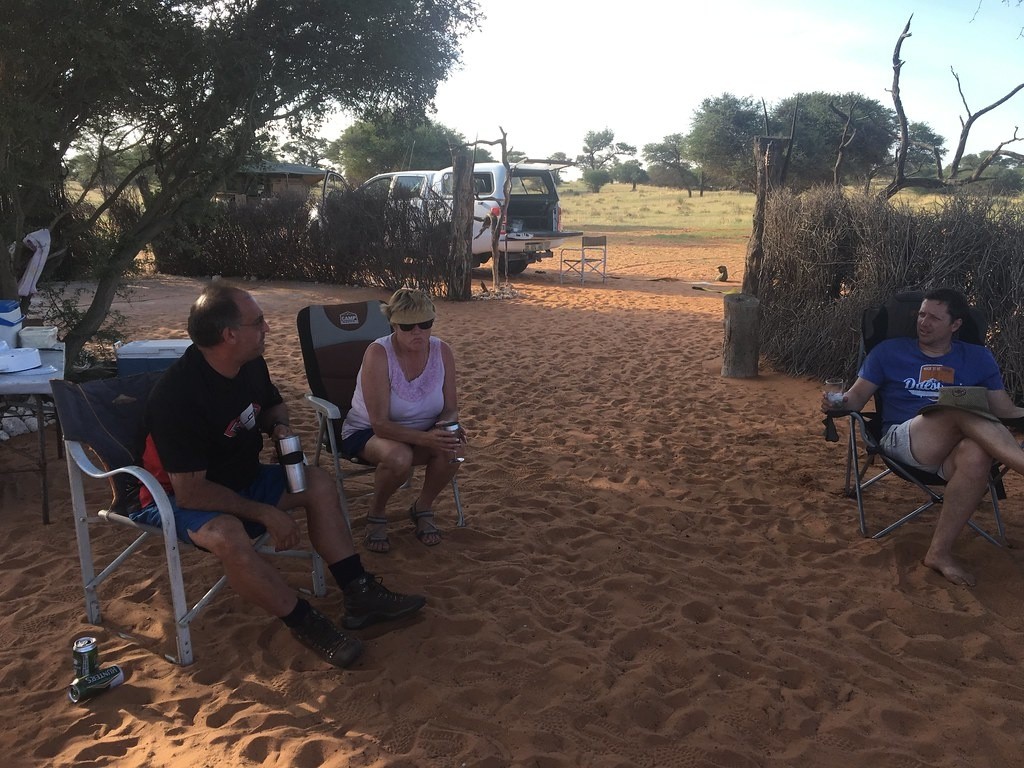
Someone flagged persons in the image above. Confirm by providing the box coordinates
[125,280,426,668]
[820,286,1024,587]
[341,288,467,553]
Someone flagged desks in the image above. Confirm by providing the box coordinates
[0,341,66,525]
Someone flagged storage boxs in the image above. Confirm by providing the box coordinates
[18,327,58,348]
[117,339,193,373]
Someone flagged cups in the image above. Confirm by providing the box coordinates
[825,378,843,411]
[275,435,306,494]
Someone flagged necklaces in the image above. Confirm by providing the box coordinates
[404,347,427,381]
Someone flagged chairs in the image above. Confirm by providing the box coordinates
[49,368,325,669]
[296,300,466,546]
[560,236,607,287]
[822,291,1024,553]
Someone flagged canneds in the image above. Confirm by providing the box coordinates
[68,664,124,703]
[72,637,99,678]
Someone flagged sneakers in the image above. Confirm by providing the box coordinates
[290,607,369,670]
[341,572,427,630]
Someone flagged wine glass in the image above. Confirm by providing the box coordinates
[441,422,465,465]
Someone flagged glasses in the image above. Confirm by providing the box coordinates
[231,315,264,331]
[398,319,434,331]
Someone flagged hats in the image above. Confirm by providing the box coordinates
[916,387,1000,422]
[390,303,436,324]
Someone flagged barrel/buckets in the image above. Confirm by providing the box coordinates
[0,300,26,349]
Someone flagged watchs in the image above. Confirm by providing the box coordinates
[269,421,289,439]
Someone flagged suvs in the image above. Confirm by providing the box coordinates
[320,157,584,277]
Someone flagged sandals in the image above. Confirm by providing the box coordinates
[408,497,442,546]
[363,511,391,553]
